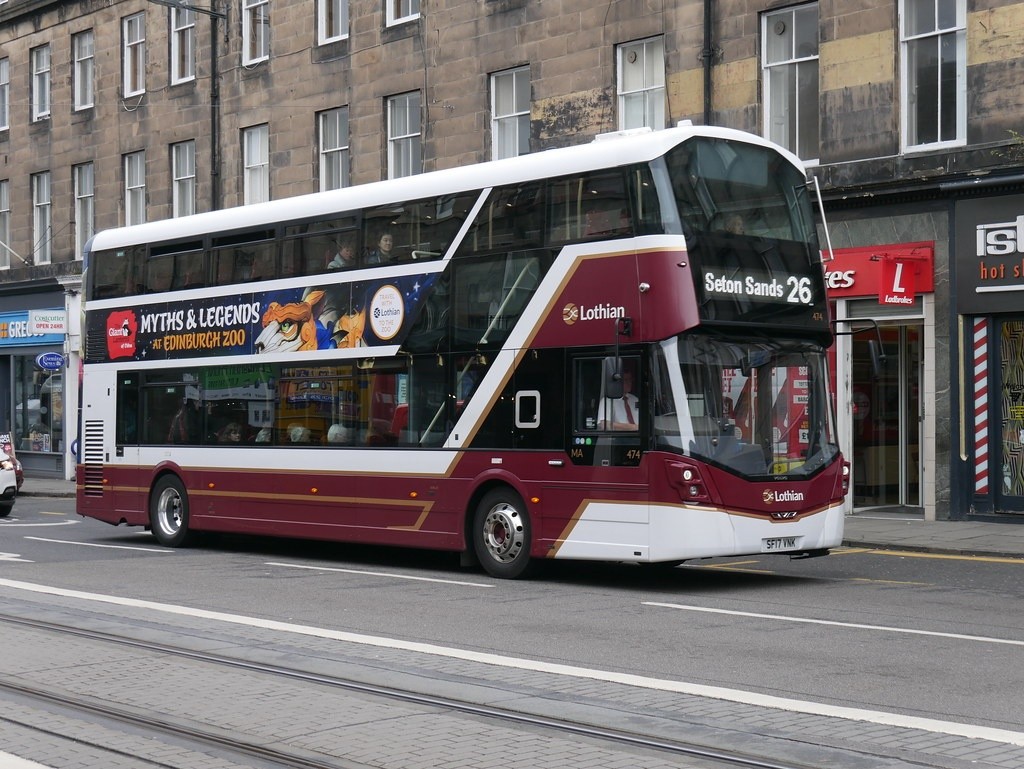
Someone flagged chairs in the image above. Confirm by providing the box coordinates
[196,434,387,443]
[145,250,335,292]
[582,209,635,238]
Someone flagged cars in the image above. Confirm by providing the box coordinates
[40,372,62,423]
[0,448,24,517]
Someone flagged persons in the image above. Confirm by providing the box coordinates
[597,370,639,430]
[366,229,399,264]
[327,242,356,271]
[724,212,745,236]
[209,421,353,444]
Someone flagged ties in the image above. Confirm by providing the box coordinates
[622,396,635,424]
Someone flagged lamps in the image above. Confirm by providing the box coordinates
[62,288,81,296]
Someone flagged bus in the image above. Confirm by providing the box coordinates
[72,118,885,580]
